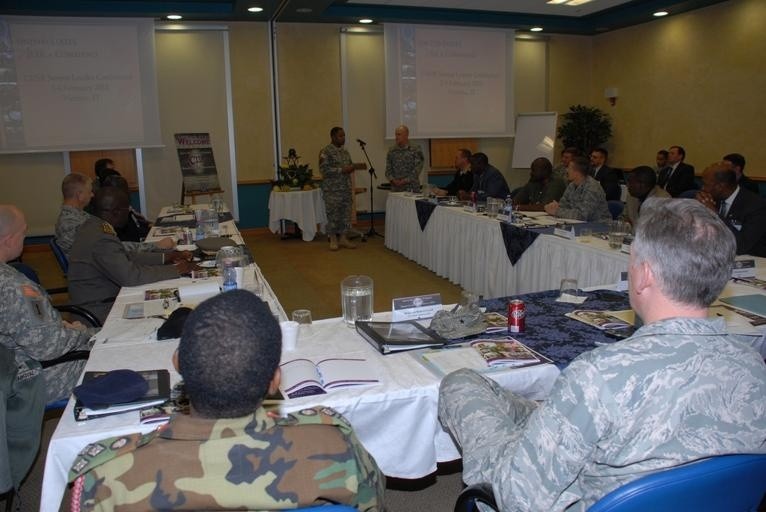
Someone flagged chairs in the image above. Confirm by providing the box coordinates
[50,235,70,278]
[456,452,766,512]
[7,266,102,411]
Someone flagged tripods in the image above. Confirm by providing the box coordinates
[360,146,385,242]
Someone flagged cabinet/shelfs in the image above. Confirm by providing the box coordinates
[69,150,139,192]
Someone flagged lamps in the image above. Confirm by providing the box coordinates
[606,87,617,106]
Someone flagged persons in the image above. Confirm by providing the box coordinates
[53,157,201,324]
[54,288,386,512]
[438,196,765,512]
[0,200,102,406]
[0,343,47,512]
[434,143,764,254]
[318,127,367,251]
[384,125,424,194]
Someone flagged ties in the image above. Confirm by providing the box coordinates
[720,201,726,218]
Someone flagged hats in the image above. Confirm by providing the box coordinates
[196,237,237,260]
[430,302,490,340]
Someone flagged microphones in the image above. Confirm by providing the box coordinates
[356,138,366,146]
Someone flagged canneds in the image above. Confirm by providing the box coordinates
[183,230,192,245]
[469,192,477,210]
[458,190,465,200]
[507,300,526,335]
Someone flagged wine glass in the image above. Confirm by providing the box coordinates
[292,310,313,358]
[184,196,192,208]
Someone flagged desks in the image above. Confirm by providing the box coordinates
[268,185,327,241]
[39,289,765,512]
[384,187,766,290]
[39,193,286,344]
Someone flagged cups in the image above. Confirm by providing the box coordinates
[560,279,577,302]
[487,197,499,217]
[581,229,591,242]
[191,271,208,282]
[341,276,374,329]
[450,196,456,204]
[422,183,431,200]
[609,221,624,249]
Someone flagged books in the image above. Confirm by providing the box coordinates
[355,318,444,355]
[563,308,632,331]
[74,369,170,421]
[277,357,380,399]
[481,311,509,333]
[179,282,221,309]
[422,338,552,377]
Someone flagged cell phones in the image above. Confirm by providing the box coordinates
[604,329,633,340]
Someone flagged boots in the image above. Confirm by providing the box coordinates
[329,234,339,250]
[339,234,358,249]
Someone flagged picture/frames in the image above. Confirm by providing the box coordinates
[512,111,558,168]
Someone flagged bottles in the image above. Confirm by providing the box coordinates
[223,261,236,293]
[208,208,219,233]
[196,224,205,241]
[212,200,224,212]
[183,226,192,244]
[505,195,512,214]
